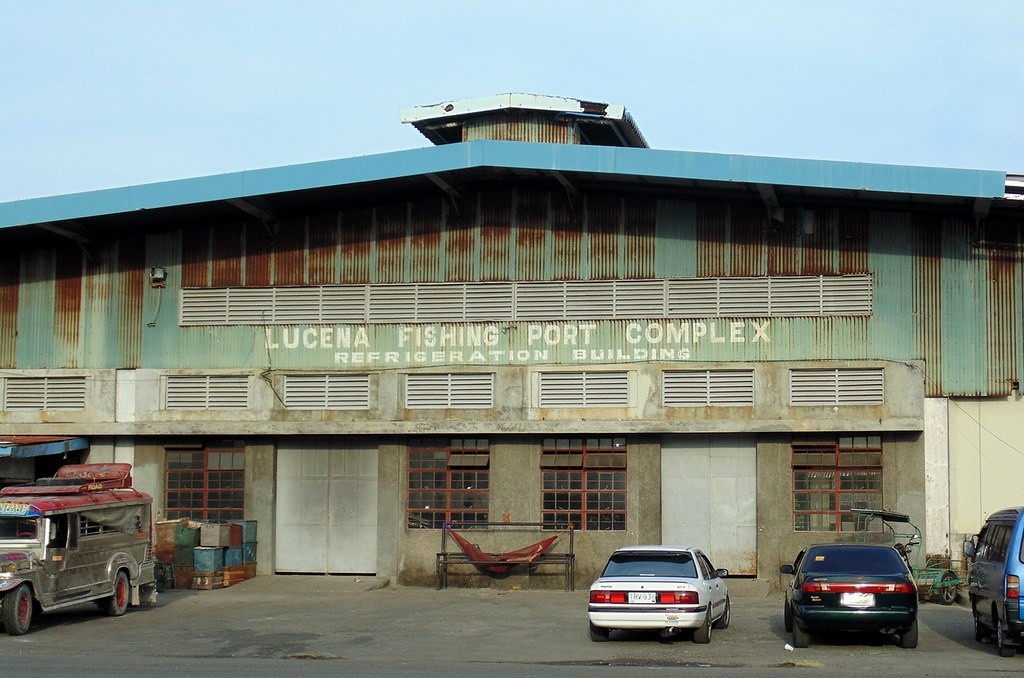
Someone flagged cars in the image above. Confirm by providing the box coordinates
[777,542,920,649]
[587,544,731,644]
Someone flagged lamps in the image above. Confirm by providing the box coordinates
[150,267,166,280]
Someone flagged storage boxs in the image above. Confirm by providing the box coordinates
[155,518,259,590]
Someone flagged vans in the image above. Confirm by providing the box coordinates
[963,505,1024,658]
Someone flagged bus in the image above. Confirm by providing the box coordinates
[0,460,156,638]
